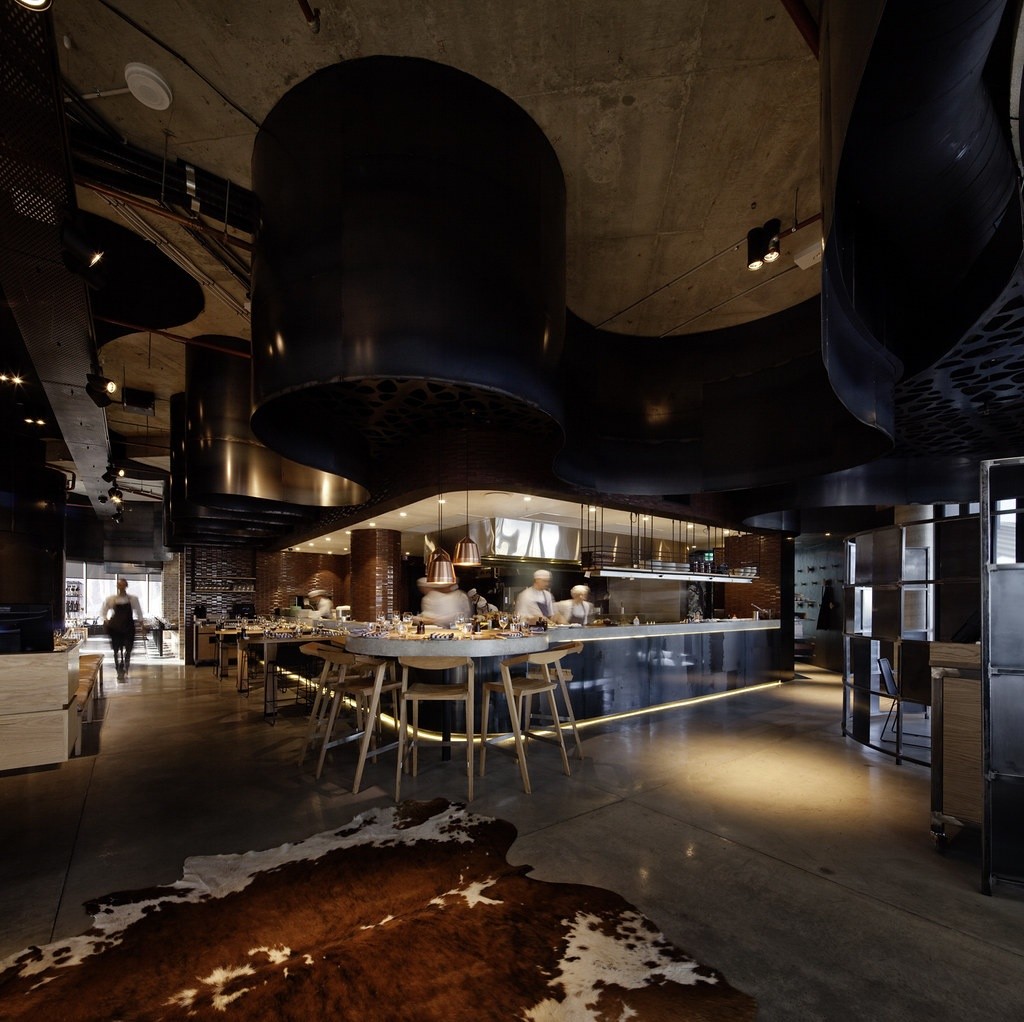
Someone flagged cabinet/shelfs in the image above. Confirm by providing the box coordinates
[193,576,256,593]
[0,644,80,772]
[580,504,762,584]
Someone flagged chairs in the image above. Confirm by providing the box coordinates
[876,658,931,750]
[211,620,586,805]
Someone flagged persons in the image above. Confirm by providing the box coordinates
[306,590,334,620]
[427,580,469,628]
[100,578,148,684]
[513,568,570,626]
[470,589,486,618]
[477,602,498,618]
[551,584,596,628]
[416,578,443,629]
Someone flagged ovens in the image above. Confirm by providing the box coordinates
[502,587,527,613]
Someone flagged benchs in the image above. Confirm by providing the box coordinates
[73,654,104,756]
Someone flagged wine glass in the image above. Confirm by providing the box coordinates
[499,612,508,635]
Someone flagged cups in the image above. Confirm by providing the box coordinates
[366,611,414,639]
[509,612,529,635]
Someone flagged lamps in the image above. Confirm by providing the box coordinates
[107,487,123,503]
[23,405,48,426]
[101,466,125,484]
[427,493,457,586]
[111,512,123,523]
[14,0,53,12]
[86,373,117,409]
[451,490,482,567]
[0,364,27,390]
[424,494,451,576]
[123,62,173,110]
[64,231,105,268]
[745,211,824,271]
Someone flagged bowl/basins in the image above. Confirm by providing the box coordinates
[638,559,691,572]
[728,566,758,576]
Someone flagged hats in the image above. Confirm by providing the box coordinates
[308,590,326,598]
[468,588,477,598]
[477,601,487,608]
[570,585,589,595]
[417,577,427,586]
[450,584,459,591]
[534,569,552,579]
